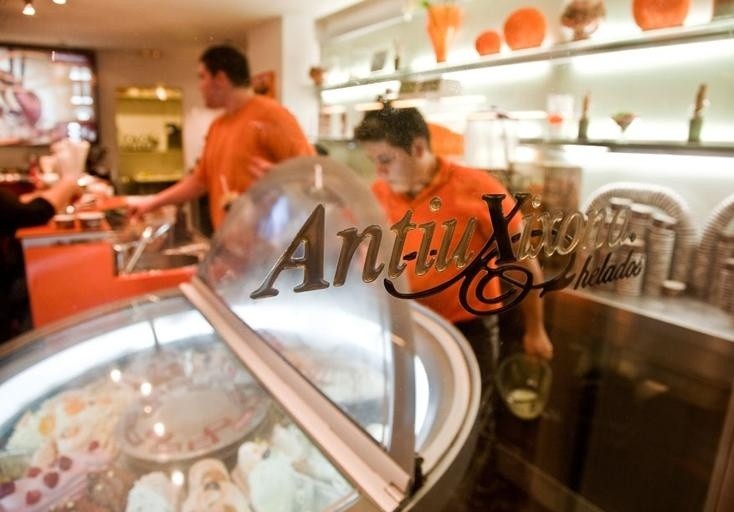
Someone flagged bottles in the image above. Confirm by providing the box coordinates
[575,88,593,140]
[688,83,708,141]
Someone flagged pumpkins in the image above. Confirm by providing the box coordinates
[504,9,546,50]
[633,0,690,30]
[476,32,501,55]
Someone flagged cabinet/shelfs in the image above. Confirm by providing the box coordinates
[314,1,733,156]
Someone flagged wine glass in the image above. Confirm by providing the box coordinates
[609,112,640,140]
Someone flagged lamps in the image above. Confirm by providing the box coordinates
[21,0,36,16]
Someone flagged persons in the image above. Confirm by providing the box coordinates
[0,170,84,348]
[342,105,553,510]
[118,43,316,269]
